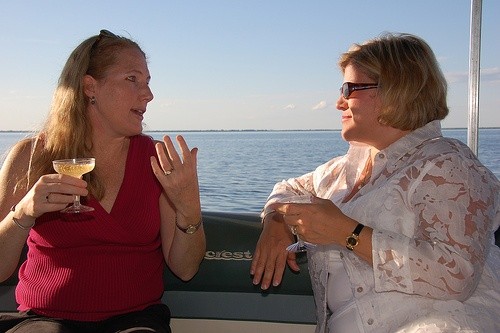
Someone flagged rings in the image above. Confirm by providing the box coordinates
[165,169,173,175]
[46,194,49,202]
[291,227,297,235]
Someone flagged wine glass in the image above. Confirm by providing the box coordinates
[53,158,96,213]
[277,194,318,252]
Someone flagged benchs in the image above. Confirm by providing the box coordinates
[0,212,317,333]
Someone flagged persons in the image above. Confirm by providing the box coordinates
[0,30,206,333]
[250,32,500,333]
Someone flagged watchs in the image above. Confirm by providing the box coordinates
[176,216,203,235]
[345,223,364,251]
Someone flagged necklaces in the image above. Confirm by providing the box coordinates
[357,158,372,189]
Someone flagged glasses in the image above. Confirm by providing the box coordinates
[338,81,383,97]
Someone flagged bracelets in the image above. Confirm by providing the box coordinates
[10,204,35,229]
